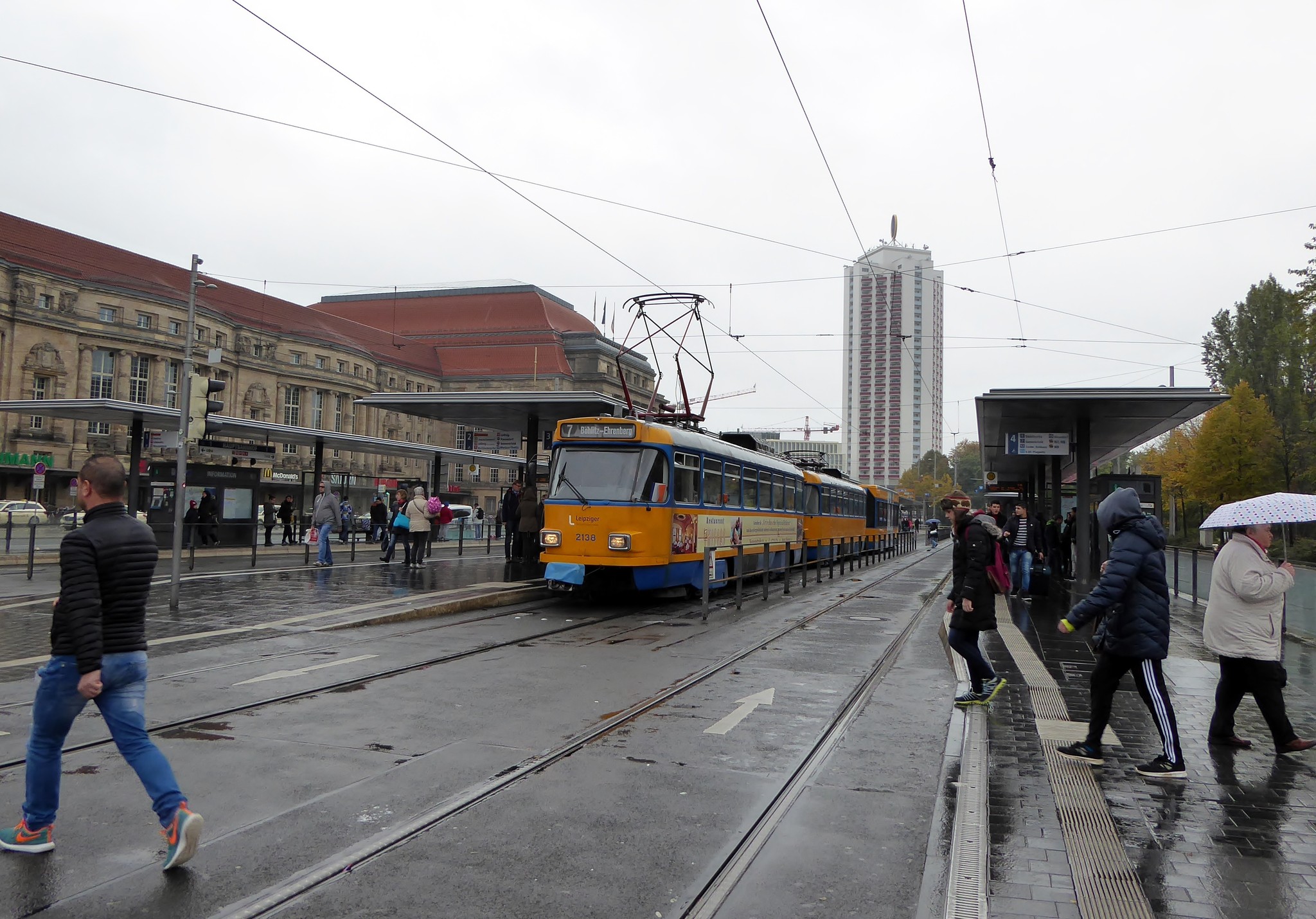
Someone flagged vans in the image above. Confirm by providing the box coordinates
[441,504,473,531]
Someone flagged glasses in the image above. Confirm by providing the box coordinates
[515,484,522,487]
[319,485,325,488]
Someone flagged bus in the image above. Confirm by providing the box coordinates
[528,292,901,599]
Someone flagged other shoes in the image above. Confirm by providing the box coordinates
[931,547,934,548]
[282,540,298,544]
[1021,594,1032,600]
[1009,584,1021,597]
[1064,576,1077,581]
[935,544,938,547]
[506,557,545,563]
[265,542,275,547]
[214,541,220,546]
[338,540,348,544]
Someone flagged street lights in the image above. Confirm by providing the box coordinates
[928,453,938,519]
[168,254,220,608]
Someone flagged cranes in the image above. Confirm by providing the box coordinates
[666,383,757,413]
[740,415,840,442]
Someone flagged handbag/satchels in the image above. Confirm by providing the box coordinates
[394,502,411,529]
[381,533,389,552]
[304,528,319,545]
[930,529,937,535]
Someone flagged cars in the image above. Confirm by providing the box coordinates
[60,505,148,530]
[257,504,282,525]
[305,508,314,516]
[0,500,50,528]
[355,511,393,529]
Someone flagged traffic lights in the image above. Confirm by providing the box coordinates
[151,464,170,482]
[188,373,225,440]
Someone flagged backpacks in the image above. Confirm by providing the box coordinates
[475,508,484,520]
[964,523,1012,596]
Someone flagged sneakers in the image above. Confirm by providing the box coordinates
[1056,741,1104,765]
[0,818,56,852]
[1135,754,1188,778]
[313,557,426,568]
[161,800,203,870]
[953,677,1007,705]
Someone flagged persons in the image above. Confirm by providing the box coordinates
[901,489,1316,777]
[181,479,547,570]
[0,454,204,872]
[732,517,742,547]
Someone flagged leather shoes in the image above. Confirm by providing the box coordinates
[1207,733,1252,746]
[1275,737,1316,754]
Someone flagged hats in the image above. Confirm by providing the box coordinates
[190,500,197,507]
[941,489,971,511]
[443,501,449,506]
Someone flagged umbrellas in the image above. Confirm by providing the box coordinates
[925,519,941,523]
[1199,492,1316,562]
